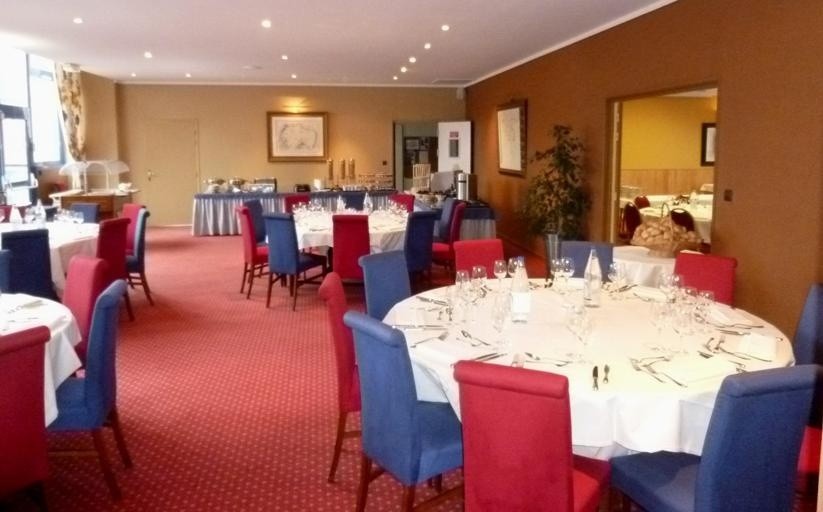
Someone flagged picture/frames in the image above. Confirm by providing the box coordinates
[493,98,528,179]
[263,110,330,164]
[699,121,715,167]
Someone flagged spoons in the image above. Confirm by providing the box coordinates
[524,351,573,365]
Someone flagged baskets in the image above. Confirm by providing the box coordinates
[630,203,703,258]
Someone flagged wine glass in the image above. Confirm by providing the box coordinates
[654,270,714,336]
[454,255,575,345]
[607,260,628,305]
[289,196,348,222]
[363,191,409,220]
[0,204,85,237]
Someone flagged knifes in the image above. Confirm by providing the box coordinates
[601,362,610,385]
[695,349,748,374]
[449,351,508,368]
[416,295,447,306]
[591,365,599,392]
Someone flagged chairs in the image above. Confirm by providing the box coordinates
[234,191,468,312]
[1,202,153,329]
[48,277,134,501]
[619,182,713,256]
[559,239,614,283]
[342,310,463,511]
[316,271,360,483]
[452,356,613,511]
[608,282,823,510]
[356,248,413,321]
[452,236,505,280]
[63,254,108,377]
[1,324,55,511]
[671,253,739,306]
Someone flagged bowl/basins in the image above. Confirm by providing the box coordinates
[412,191,457,201]
[201,177,248,185]
[313,178,325,190]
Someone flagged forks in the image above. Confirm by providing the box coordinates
[406,330,450,348]
[714,334,727,350]
[629,352,686,388]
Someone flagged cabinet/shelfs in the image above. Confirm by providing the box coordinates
[60,189,139,220]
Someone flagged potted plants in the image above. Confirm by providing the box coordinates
[512,118,595,276]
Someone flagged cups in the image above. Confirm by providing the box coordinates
[341,184,364,192]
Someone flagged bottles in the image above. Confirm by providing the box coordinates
[582,245,603,307]
[325,157,356,181]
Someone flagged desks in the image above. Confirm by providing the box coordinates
[587,244,702,286]
[381,273,786,463]
[1,291,84,426]
[192,191,396,237]
